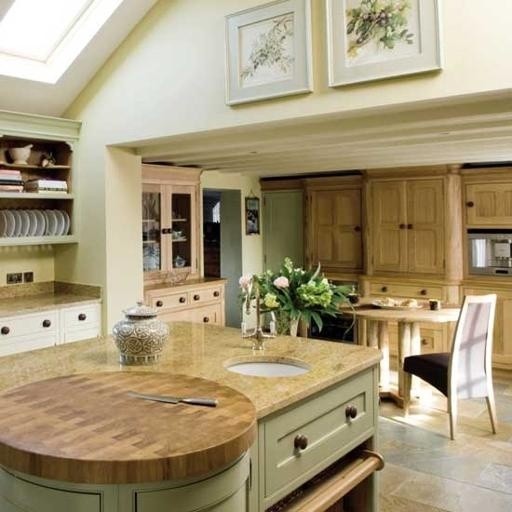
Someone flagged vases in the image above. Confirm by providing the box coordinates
[272,311,301,336]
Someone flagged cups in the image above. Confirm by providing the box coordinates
[429,300,441,312]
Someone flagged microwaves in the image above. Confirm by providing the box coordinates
[468,233,512,276]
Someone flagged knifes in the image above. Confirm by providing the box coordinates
[127,388,219,410]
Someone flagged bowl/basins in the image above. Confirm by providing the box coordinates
[172,230,183,239]
[9,148,32,164]
[143,229,159,240]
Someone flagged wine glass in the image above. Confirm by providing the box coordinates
[143,199,155,218]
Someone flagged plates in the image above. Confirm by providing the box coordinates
[373,298,424,311]
[0,209,71,239]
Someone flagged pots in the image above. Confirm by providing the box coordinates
[172,252,189,268]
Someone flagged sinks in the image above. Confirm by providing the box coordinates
[223,354,312,378]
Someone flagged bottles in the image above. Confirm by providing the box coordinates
[112,299,169,368]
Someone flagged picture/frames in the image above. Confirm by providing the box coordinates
[245,196,260,236]
[225,1,314,107]
[323,0,443,87]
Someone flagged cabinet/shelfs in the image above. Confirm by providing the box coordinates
[364,168,460,271]
[255,364,384,512]
[304,173,364,297]
[462,167,512,231]
[144,278,228,326]
[0,110,82,247]
[0,298,103,361]
[367,281,443,371]
[141,162,204,282]
[461,278,512,372]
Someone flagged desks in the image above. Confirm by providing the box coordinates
[334,298,461,407]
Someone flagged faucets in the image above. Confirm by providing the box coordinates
[245,276,260,328]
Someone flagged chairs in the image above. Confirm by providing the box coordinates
[403,294,498,438]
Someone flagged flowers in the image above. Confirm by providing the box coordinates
[236,258,350,331]
